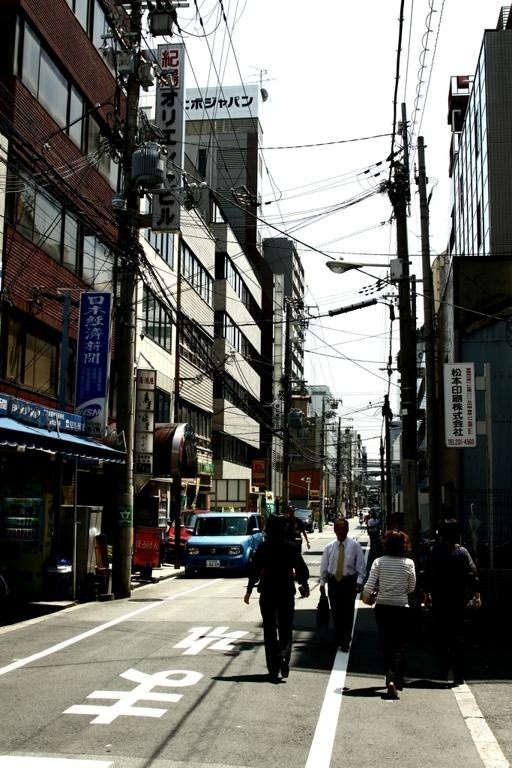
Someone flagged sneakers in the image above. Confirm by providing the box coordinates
[268,658,289,678]
[387,681,398,698]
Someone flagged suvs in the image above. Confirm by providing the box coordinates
[183,512,263,575]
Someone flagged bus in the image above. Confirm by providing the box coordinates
[307,499,321,522]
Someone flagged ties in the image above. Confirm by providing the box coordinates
[336,543,345,583]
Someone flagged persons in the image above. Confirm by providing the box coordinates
[319,518,367,651]
[423,517,484,686]
[362,529,417,699]
[243,512,312,680]
[286,504,311,554]
[363,507,382,546]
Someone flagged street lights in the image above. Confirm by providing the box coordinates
[301,477,312,510]
[322,253,426,627]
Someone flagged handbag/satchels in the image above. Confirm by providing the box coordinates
[318,594,331,622]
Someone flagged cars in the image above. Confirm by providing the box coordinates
[354,506,380,521]
[293,509,317,534]
[163,508,206,560]
